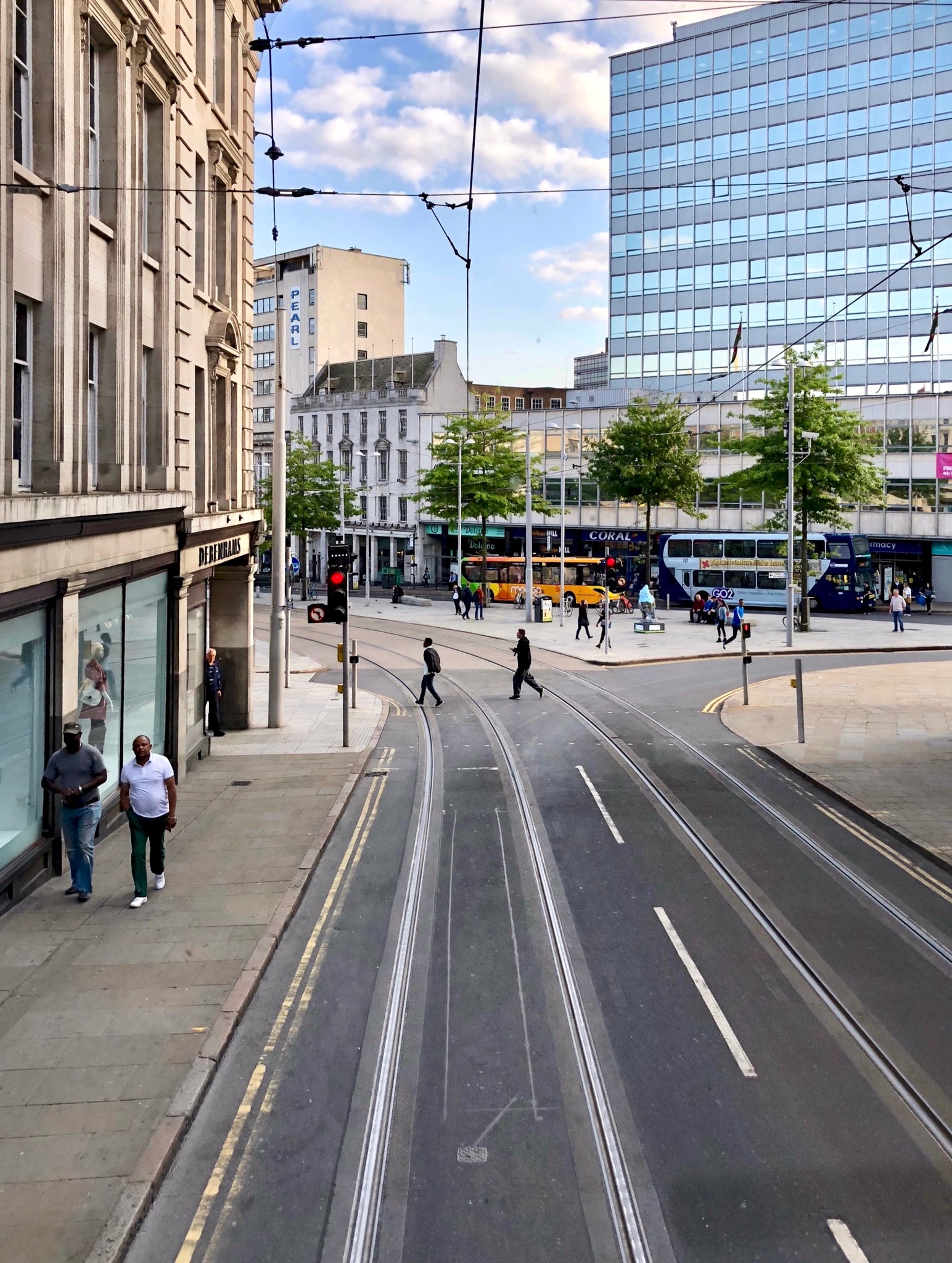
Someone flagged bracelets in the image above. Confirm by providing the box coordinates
[168,816,176,819]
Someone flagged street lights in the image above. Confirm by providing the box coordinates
[545,421,582,623]
[354,451,381,607]
[770,357,812,644]
[445,434,476,589]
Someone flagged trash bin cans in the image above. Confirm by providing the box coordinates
[349,572,359,590]
[534,594,553,622]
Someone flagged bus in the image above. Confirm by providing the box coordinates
[458,555,624,607]
[658,531,879,613]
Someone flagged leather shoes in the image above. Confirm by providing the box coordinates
[79,896,89,903]
[65,887,78,895]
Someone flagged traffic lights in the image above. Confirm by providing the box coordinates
[605,556,618,587]
[598,563,605,574]
[327,567,348,624]
[263,547,272,571]
[327,544,350,565]
[742,622,751,638]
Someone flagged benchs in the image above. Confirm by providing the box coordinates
[726,616,757,627]
[397,595,432,606]
[435,578,450,590]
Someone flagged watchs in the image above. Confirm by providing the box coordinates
[78,785,83,793]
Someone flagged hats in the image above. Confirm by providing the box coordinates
[63,721,81,734]
[711,596,716,599]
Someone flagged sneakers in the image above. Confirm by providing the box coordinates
[540,687,544,698]
[723,641,726,649]
[509,695,521,700]
[130,896,147,908]
[596,644,601,648]
[434,700,444,707]
[415,701,424,705]
[588,636,593,639]
[576,638,580,640]
[155,871,166,890]
[214,731,225,736]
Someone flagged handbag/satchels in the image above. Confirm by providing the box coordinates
[609,622,612,628]
[78,678,102,706]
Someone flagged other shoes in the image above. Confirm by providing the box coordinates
[723,638,727,642]
[455,611,484,621]
[716,638,721,642]
[863,611,932,631]
[689,619,719,626]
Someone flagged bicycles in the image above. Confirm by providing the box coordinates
[595,593,614,615]
[783,612,802,632]
[555,594,573,617]
[307,581,318,601]
[514,590,526,609]
[612,592,634,614]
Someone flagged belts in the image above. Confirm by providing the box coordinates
[63,795,100,810]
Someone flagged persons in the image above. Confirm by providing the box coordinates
[119,736,177,907]
[448,571,456,591]
[390,584,404,604]
[97,632,119,700]
[38,722,107,905]
[415,637,445,707]
[723,599,749,651]
[863,582,870,615]
[715,600,727,643]
[450,582,486,620]
[76,642,114,757]
[422,567,430,588]
[923,582,935,615]
[688,594,730,625]
[8,641,34,698]
[204,646,227,737]
[508,629,544,699]
[595,606,612,649]
[575,598,594,640]
[889,577,911,632]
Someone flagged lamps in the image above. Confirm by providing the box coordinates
[382,523,387,527]
[369,522,374,527]
[351,521,356,525]
[395,524,400,528]
[404,524,407,528]
[358,521,362,526]
[412,524,417,529]
[363,522,368,526]
[376,523,380,527]
[389,523,393,528]
